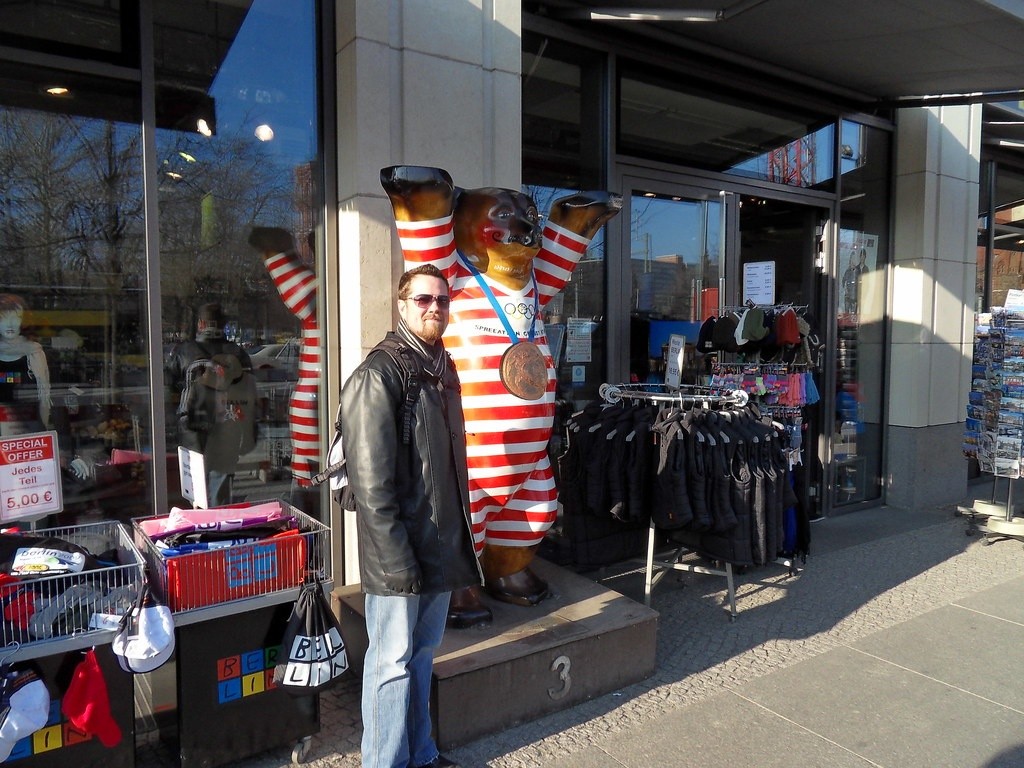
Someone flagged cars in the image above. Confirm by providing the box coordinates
[250,343,301,383]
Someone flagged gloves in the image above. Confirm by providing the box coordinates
[709,368,821,409]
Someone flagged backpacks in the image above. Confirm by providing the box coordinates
[174,340,257,454]
[311,337,421,511]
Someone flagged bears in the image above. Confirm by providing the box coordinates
[378,164,625,632]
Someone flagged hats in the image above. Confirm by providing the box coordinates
[61,651,121,749]
[112,584,175,674]
[696,306,822,369]
[0,672,51,764]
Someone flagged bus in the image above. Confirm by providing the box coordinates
[0,285,149,381]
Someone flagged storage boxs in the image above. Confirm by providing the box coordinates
[135,502,306,612]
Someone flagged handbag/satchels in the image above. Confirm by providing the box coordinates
[271,581,351,698]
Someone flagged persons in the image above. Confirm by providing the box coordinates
[341,265,486,768]
[168,303,260,509]
[0,293,54,436]
[841,249,868,315]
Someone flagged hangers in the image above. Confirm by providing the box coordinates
[567,383,784,445]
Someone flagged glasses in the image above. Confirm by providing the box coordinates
[401,294,452,310]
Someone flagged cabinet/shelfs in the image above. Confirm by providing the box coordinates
[0,497,334,768]
